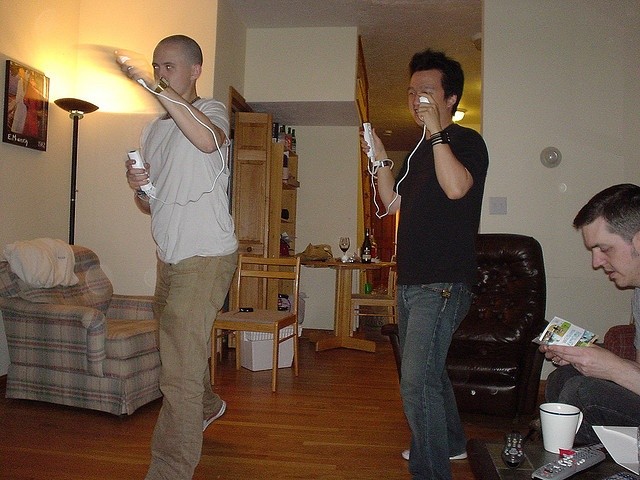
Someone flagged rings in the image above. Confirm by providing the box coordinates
[552,359,558,364]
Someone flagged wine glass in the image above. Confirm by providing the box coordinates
[339,237,350,256]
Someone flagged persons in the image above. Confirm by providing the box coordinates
[358,50,490,480]
[11,67,27,134]
[23,74,43,139]
[538,183,640,446]
[115,34,239,480]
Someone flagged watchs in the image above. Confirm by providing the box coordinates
[160,76,170,87]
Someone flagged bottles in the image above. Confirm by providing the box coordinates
[278,125,296,154]
[361,228,371,264]
[370,229,379,259]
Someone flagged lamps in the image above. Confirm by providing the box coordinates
[54,94,98,245]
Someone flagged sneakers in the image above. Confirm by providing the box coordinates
[202,400,227,432]
[401,447,468,460]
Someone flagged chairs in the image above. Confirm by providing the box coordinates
[210,255,302,391]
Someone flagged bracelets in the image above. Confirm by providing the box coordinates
[375,159,391,168]
[135,190,146,196]
[430,131,451,146]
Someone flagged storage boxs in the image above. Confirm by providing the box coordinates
[238,325,302,372]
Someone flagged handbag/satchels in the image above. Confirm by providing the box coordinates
[296,243,333,262]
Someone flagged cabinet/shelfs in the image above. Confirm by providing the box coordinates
[267,145,299,312]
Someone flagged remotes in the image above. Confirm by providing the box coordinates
[531,448,605,480]
[501,432,526,469]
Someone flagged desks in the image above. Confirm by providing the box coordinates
[296,253,396,352]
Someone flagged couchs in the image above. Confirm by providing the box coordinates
[0,245,164,415]
[382,235,548,424]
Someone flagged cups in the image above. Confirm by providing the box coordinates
[539,403,584,455]
